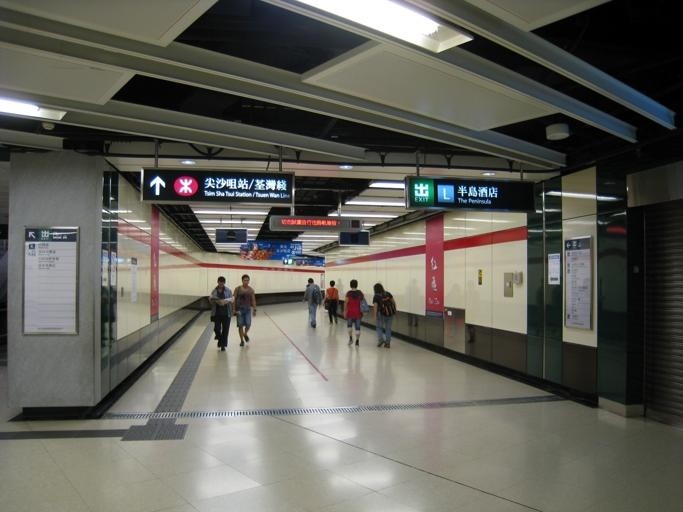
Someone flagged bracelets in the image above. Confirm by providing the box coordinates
[254,309,256,312]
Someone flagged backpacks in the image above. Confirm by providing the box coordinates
[312,284,320,303]
[377,292,396,316]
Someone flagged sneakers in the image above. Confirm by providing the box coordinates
[217,340,226,351]
[377,341,390,348]
[348,340,359,346]
[239,335,249,346]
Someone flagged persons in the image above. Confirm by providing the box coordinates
[323,280,339,325]
[302,278,321,328]
[210,277,232,351]
[373,283,396,348]
[233,275,257,346]
[344,279,364,347]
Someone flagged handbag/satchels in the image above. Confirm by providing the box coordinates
[325,299,330,309]
[360,299,370,314]
